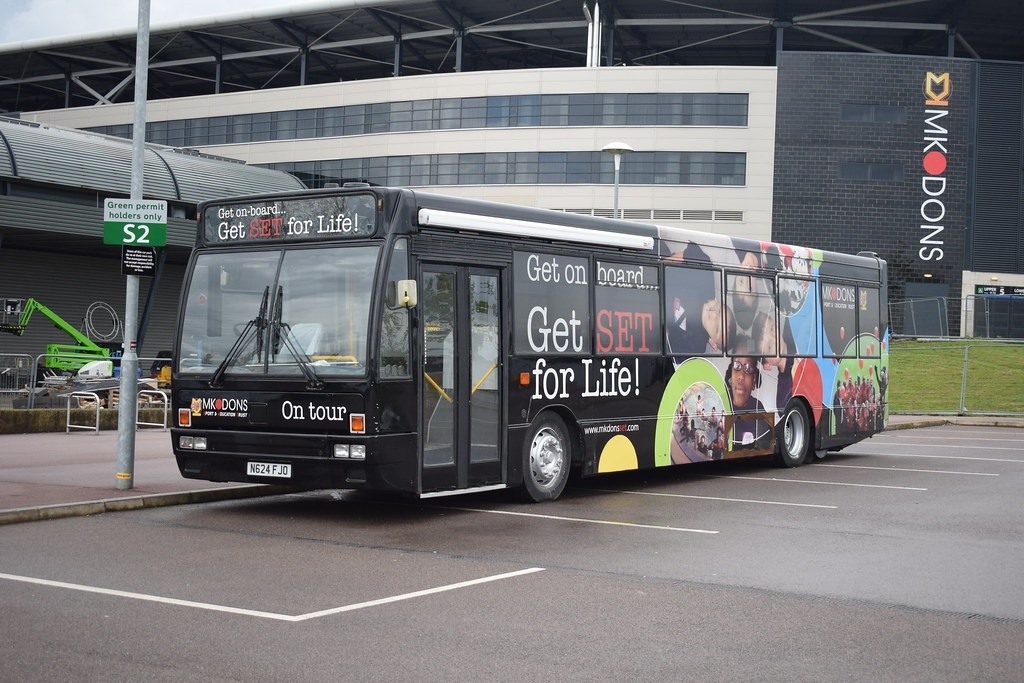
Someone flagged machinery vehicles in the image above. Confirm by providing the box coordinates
[0,297,112,383]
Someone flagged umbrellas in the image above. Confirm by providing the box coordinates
[874,326,879,337]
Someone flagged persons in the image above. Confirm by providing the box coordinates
[658,236,805,460]
[833,365,889,434]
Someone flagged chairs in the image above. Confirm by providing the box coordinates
[280,323,326,356]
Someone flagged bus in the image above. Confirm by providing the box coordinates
[155,178,890,503]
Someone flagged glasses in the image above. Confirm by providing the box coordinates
[731,362,757,374]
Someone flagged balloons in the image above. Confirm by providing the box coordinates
[840,326,845,339]
[881,340,886,351]
[844,368,849,380]
[866,342,875,357]
[832,353,836,365]
[859,359,863,369]
[869,365,872,377]
